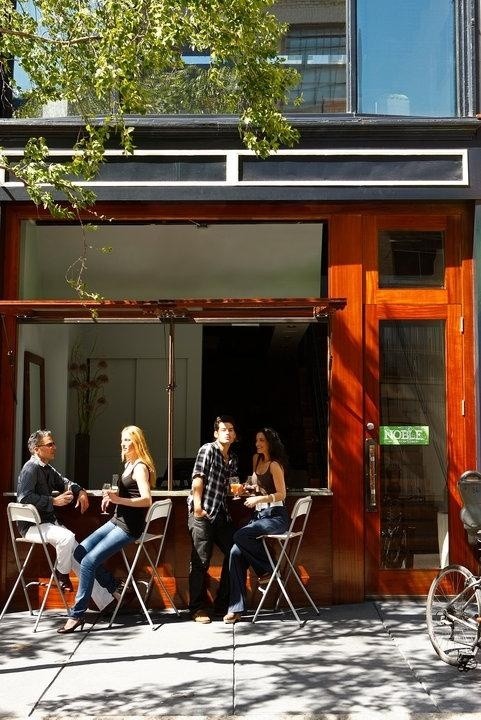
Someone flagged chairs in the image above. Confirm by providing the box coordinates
[0,502,71,633]
[105,499,182,632]
[252,495,321,627]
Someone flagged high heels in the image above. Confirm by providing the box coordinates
[57,616,84,634]
[114,580,129,608]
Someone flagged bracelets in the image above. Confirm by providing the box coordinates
[270,493,275,502]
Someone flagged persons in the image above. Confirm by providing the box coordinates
[222,429,286,625]
[56,425,157,633]
[15,429,115,612]
[186,415,240,624]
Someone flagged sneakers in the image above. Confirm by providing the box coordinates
[223,612,241,624]
[214,606,227,617]
[193,610,211,624]
[259,569,281,584]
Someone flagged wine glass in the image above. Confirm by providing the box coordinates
[100,482,112,515]
[229,475,243,500]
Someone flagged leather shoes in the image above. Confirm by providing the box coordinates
[102,599,124,617]
[54,569,73,592]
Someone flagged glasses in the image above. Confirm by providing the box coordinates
[36,442,58,449]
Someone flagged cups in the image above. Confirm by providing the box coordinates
[64,482,72,491]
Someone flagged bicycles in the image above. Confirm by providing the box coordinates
[425,534,481,670]
